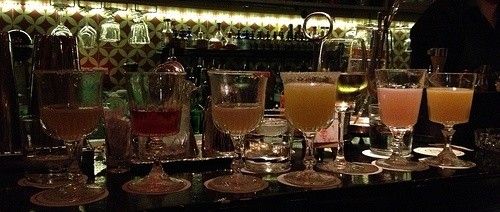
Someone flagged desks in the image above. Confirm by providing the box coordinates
[0,151,500,212]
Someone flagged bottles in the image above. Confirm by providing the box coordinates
[162,18,332,51]
[0,31,29,161]
[185,56,314,135]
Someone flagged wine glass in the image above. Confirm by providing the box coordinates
[124,69,187,193]
[206,68,270,192]
[318,38,379,174]
[373,67,431,171]
[425,72,478,168]
[51,4,151,49]
[31,68,107,203]
[279,70,341,188]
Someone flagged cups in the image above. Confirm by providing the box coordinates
[133,80,197,162]
[201,95,246,157]
[348,27,394,99]
[243,108,292,172]
[101,107,131,174]
[27,34,80,115]
[367,103,413,156]
[18,112,72,184]
